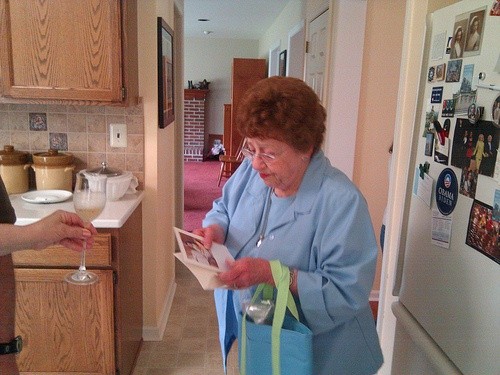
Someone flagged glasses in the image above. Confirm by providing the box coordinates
[240,145,294,164]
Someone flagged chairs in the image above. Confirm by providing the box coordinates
[217,135,248,187]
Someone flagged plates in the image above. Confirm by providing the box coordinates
[20,189,73,204]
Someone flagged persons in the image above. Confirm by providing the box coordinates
[0,175,97,375]
[192,76,384,375]
[450,16,495,196]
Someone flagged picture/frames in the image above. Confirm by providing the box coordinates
[278,50,286,77]
[157,16,175,129]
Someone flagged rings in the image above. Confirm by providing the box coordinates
[234,283,239,288]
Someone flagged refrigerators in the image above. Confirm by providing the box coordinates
[389,0,500,375]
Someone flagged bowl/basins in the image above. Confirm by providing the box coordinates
[81,169,133,201]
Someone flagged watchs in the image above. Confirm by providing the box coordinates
[0,335,23,354]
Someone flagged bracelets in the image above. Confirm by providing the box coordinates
[289,267,295,289]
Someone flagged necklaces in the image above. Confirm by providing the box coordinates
[256,187,274,247]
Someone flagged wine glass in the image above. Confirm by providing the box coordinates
[63,172,108,286]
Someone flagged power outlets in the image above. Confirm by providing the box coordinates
[109,124,128,148]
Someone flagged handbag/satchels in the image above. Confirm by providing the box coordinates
[237,260,314,375]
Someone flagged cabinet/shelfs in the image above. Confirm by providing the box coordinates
[0,0,139,109]
[3,187,143,375]
[223,57,267,177]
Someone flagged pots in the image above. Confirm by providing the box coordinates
[0,145,32,194]
[30,148,76,192]
